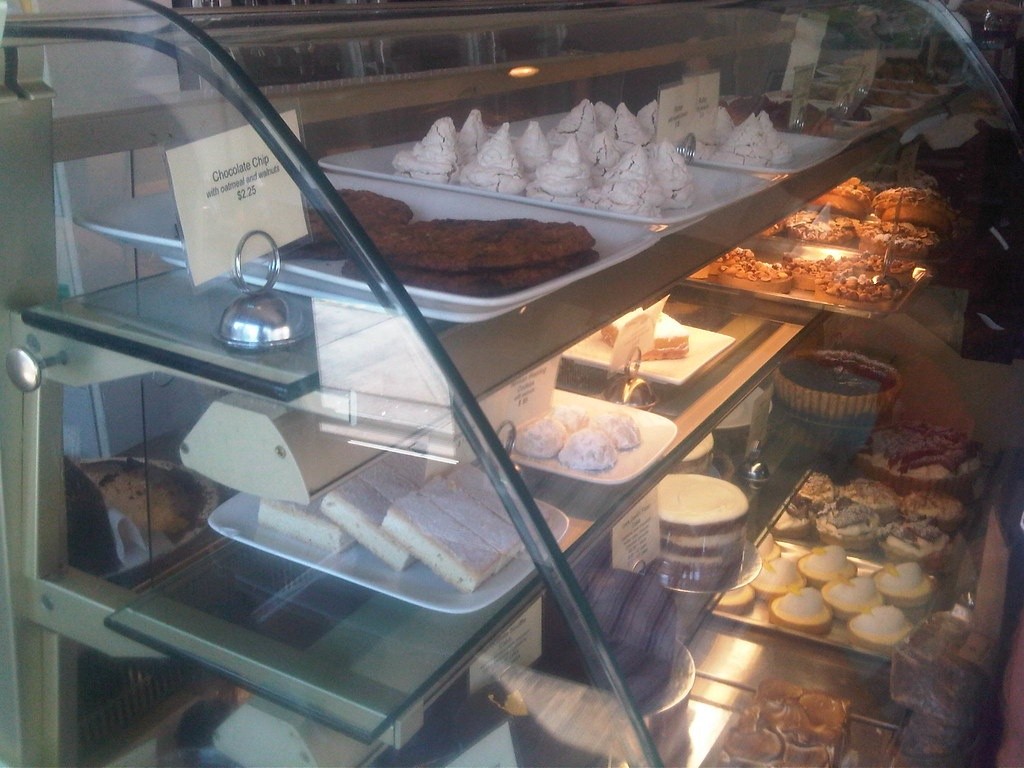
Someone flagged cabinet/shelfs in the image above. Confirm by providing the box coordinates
[0,0,1024,768]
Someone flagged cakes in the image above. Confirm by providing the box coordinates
[773,350,903,417]
[646,430,747,576]
[72,458,204,532]
[602,306,690,361]
[703,246,916,316]
[764,178,964,262]
[850,423,988,497]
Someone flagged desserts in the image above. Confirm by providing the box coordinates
[713,472,970,651]
[393,71,932,218]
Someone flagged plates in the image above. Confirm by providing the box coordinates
[208,463,569,615]
[561,308,736,386]
[70,57,967,308]
[509,388,678,484]
[664,539,762,593]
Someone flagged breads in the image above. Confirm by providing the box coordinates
[256,452,551,597]
[515,404,642,472]
[176,188,603,301]
